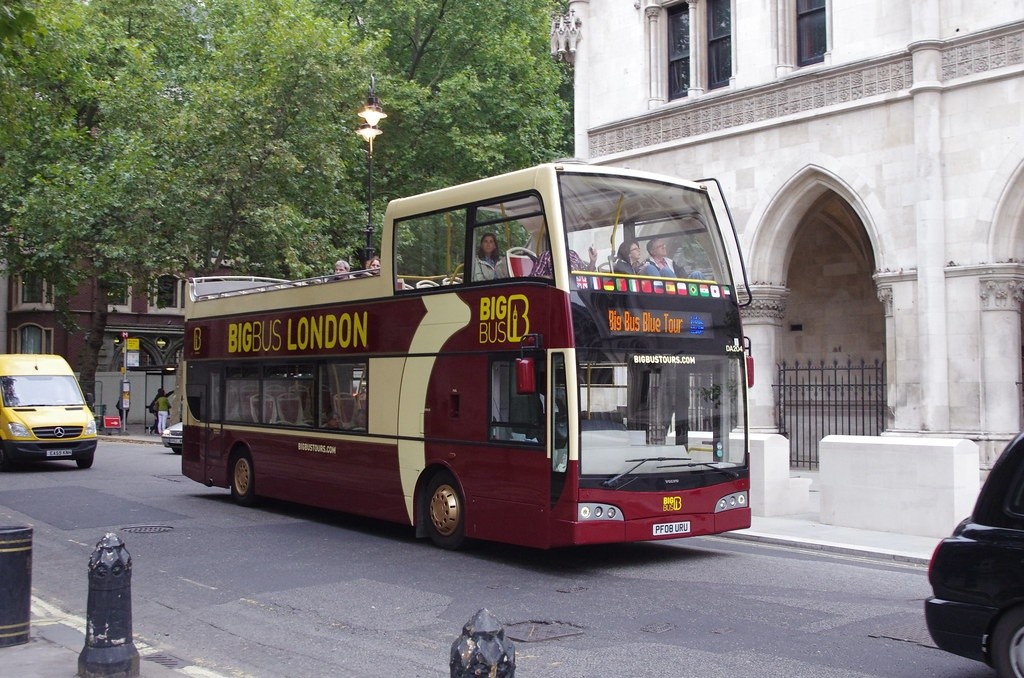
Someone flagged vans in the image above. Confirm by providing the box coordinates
[0,354,98,473]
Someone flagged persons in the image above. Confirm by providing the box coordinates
[327,410,344,430]
[116,395,130,430]
[642,239,706,280]
[473,234,507,281]
[614,240,661,276]
[333,259,351,281]
[149,388,174,436]
[529,226,597,277]
[365,256,381,277]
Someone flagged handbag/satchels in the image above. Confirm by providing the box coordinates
[153,403,159,411]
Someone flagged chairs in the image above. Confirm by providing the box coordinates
[217,382,368,431]
[506,246,538,278]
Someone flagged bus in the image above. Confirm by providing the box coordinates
[181,161,755,550]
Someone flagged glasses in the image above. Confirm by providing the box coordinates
[629,247,640,252]
[653,243,667,249]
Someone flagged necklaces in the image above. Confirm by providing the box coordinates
[657,258,665,262]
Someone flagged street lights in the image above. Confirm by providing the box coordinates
[355,75,386,263]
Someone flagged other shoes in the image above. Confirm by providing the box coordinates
[155,431,159,435]
[149,429,152,435]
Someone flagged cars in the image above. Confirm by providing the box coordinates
[161,421,183,454]
[924,432,1024,678]
